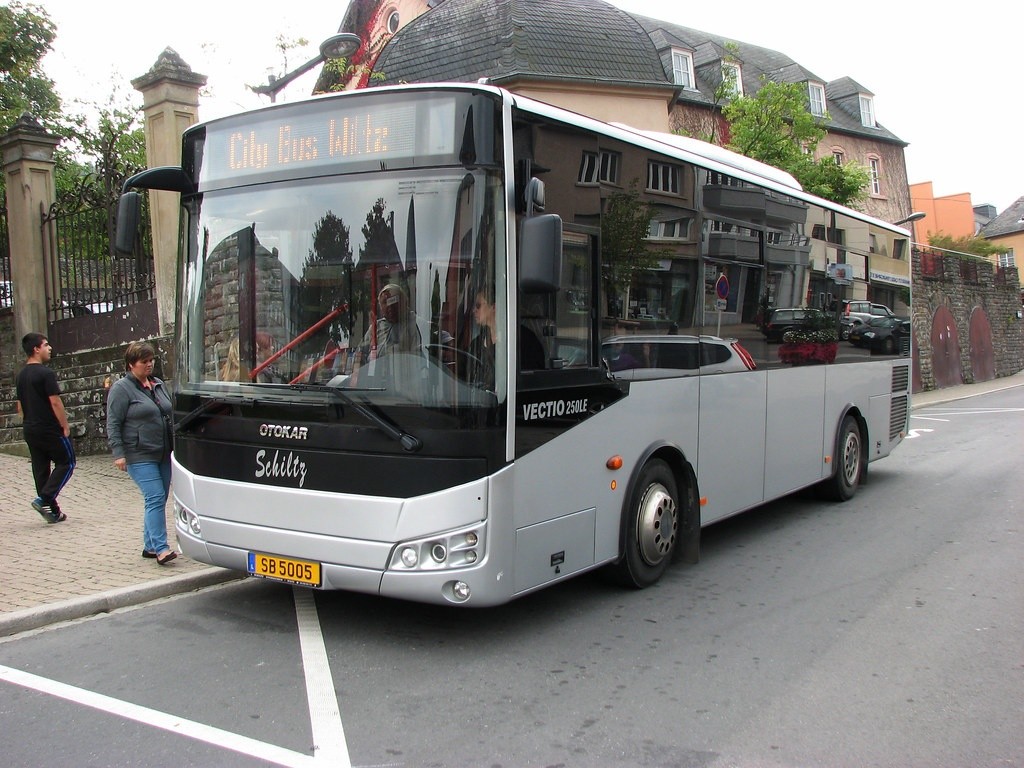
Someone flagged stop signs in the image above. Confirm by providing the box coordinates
[715,275,731,301]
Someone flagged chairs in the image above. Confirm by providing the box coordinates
[518,291,555,366]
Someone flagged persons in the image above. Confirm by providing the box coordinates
[601,309,651,371]
[220,335,262,393]
[445,278,546,393]
[333,390,345,419]
[255,332,274,384]
[351,284,429,388]
[664,289,717,369]
[106,342,178,564]
[16,332,77,524]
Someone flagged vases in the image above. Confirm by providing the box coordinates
[792,359,823,366]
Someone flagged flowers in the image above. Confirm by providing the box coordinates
[777,306,838,367]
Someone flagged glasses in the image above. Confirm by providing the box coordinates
[473,303,492,309]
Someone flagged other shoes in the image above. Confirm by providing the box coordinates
[142,550,157,558]
[157,552,176,564]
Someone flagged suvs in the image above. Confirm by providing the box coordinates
[567,335,757,379]
[759,304,837,343]
[824,299,897,340]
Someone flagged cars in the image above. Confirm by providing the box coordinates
[851,315,910,355]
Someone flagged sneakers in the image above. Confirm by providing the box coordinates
[32,497,66,524]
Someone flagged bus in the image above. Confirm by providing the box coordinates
[112,83,913,609]
